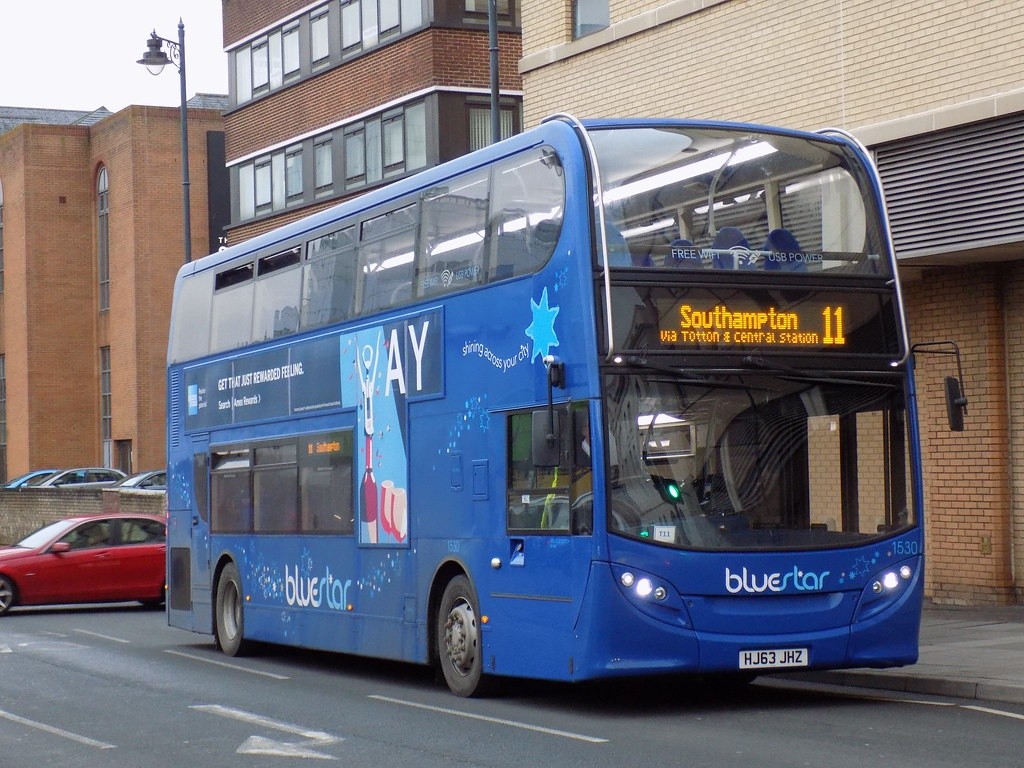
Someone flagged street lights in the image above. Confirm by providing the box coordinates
[135,17,193,265]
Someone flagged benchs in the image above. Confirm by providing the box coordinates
[473,232,553,282]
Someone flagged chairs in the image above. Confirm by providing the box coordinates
[87,525,106,548]
[664,240,704,268]
[629,248,656,267]
[391,282,412,305]
[764,229,807,272]
[712,227,758,270]
[594,221,633,268]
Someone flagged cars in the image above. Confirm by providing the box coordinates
[0,469,87,488]
[28,467,127,490]
[0,510,166,613]
[31,468,126,490]
[109,469,168,490]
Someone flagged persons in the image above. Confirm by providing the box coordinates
[574,415,592,468]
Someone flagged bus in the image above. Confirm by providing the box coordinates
[166,110,968,700]
